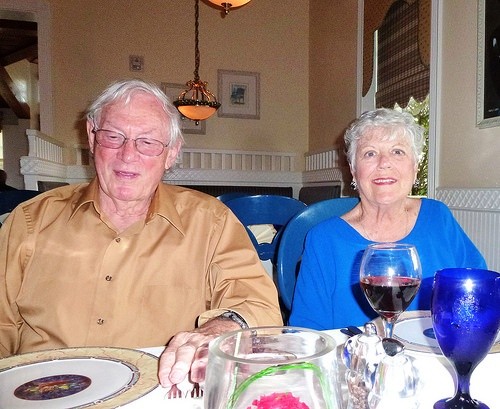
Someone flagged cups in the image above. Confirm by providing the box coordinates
[203,326,342,409]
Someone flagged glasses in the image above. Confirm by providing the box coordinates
[95,129,173,157]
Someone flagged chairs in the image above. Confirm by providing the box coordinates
[216,193,361,311]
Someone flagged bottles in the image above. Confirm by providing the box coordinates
[368,338,424,409]
[342,322,384,409]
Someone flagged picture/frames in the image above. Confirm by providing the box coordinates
[475,0,500,129]
[217,69,261,119]
[161,82,206,135]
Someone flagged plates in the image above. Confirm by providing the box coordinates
[394,310,500,353]
[0,347,161,409]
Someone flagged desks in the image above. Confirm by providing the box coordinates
[116,327,500,409]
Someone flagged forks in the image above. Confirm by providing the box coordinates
[185,382,203,399]
[163,383,184,400]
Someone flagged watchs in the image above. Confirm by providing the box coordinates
[220,309,251,330]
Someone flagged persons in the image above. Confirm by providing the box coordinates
[284,106,489,333]
[0,80,284,388]
[0,170,22,215]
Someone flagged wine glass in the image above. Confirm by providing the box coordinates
[431,268,500,409]
[360,243,422,355]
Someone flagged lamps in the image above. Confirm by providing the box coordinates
[208,0,251,14]
[174,0,222,125]
